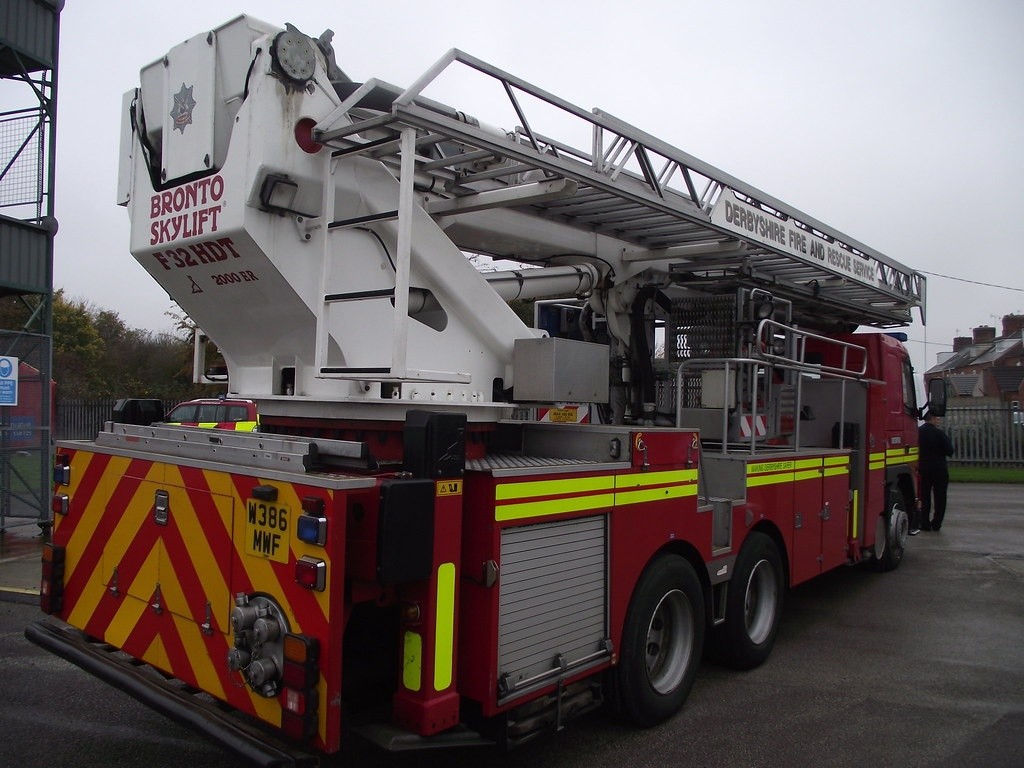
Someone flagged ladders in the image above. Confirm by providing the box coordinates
[94,421,378,473]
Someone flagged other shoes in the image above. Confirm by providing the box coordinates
[921,524,931,531]
[930,520,940,532]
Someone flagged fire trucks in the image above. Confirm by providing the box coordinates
[20,15,949,768]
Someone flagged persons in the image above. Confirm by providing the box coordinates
[919,416,954,530]
